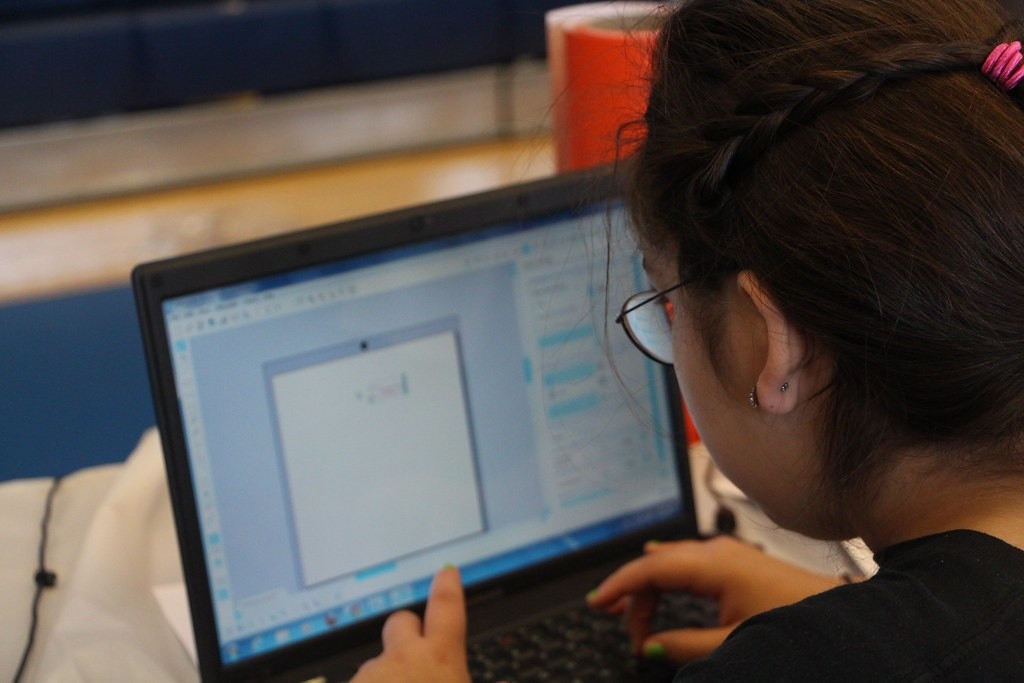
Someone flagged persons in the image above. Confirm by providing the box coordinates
[350,1,1024,682]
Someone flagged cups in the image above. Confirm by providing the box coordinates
[546,2,680,172]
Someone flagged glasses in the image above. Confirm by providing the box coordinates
[615,279,691,367]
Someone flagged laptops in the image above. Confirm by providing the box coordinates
[130,159,727,683]
